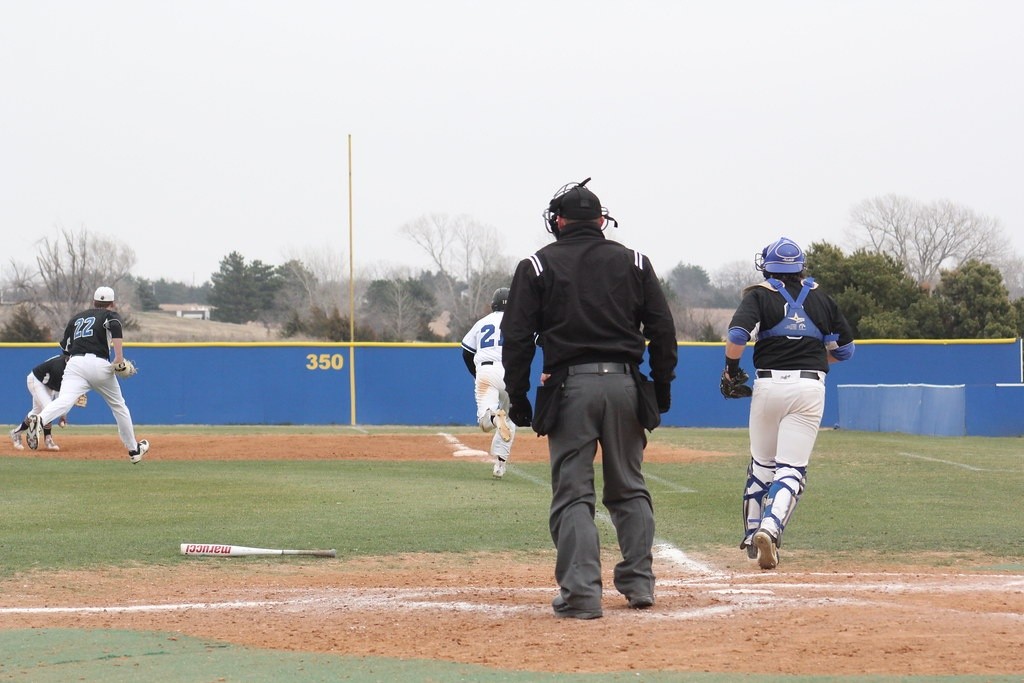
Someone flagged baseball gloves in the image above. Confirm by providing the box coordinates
[73,394,88,406]
[112,359,137,377]
[720,367,753,399]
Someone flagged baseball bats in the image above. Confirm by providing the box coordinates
[180,543,337,558]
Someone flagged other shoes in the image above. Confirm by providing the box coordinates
[748,544,758,560]
[493,465,507,479]
[752,529,779,570]
[496,409,511,442]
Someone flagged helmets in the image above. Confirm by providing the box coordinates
[491,288,510,311]
[94,286,115,302]
[755,237,808,272]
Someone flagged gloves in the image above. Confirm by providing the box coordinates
[509,398,532,427]
[656,381,670,412]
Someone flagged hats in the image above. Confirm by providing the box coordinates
[543,177,608,238]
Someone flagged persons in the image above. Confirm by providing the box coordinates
[720,237,855,570]
[460,288,516,477]
[499,182,678,620]
[10,354,87,451]
[26,286,150,463]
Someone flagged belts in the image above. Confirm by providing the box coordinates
[482,362,493,365]
[72,353,102,358]
[756,371,820,380]
[568,362,627,373]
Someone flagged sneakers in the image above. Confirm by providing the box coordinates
[26,414,40,450]
[129,439,149,464]
[10,429,24,450]
[552,596,603,619]
[628,595,653,608]
[45,437,60,451]
